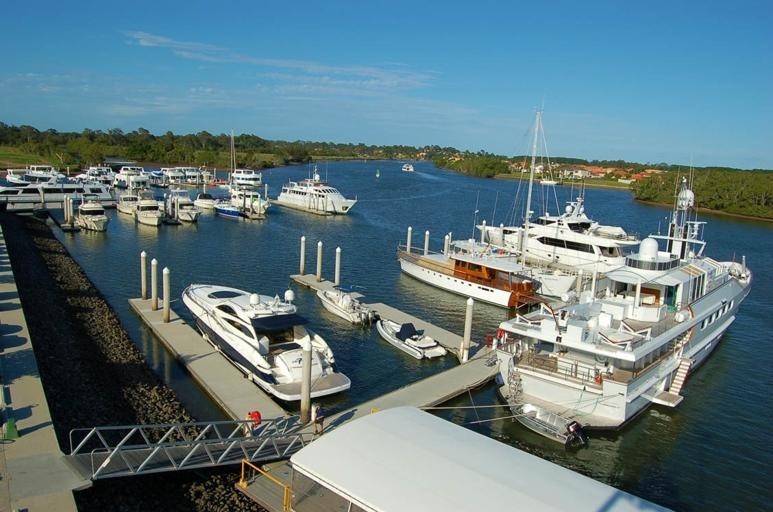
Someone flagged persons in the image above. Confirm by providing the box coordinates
[313,403,324,435]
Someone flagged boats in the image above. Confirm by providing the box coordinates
[312,283,373,327]
[483,163,756,441]
[375,314,447,362]
[0,143,272,231]
[505,403,584,447]
[179,274,353,404]
[538,178,561,186]
[400,162,414,172]
[275,157,361,216]
